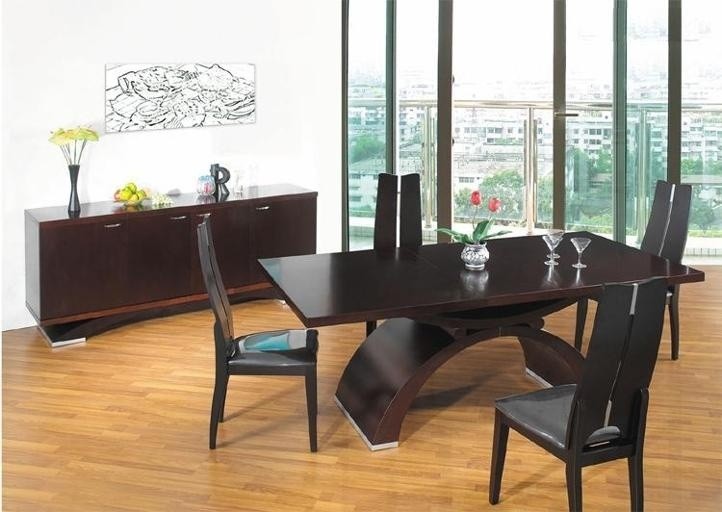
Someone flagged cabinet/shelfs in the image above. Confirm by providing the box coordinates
[23,181,319,350]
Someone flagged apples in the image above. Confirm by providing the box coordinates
[114,182,147,202]
[111,205,148,212]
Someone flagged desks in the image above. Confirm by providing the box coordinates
[256,230,705,450]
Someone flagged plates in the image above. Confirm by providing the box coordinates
[114,198,150,206]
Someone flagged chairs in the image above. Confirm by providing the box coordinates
[574,179,692,361]
[366,172,423,339]
[488,276,669,512]
[195,211,320,453]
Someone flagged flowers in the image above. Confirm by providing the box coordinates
[433,190,513,244]
[46,125,99,165]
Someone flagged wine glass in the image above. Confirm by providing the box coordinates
[540,264,587,290]
[542,228,591,269]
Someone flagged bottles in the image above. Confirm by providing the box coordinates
[196,176,216,195]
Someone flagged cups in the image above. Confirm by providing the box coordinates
[233,180,243,194]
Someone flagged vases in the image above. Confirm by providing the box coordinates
[68,165,81,214]
[460,271,489,291]
[459,244,490,271]
